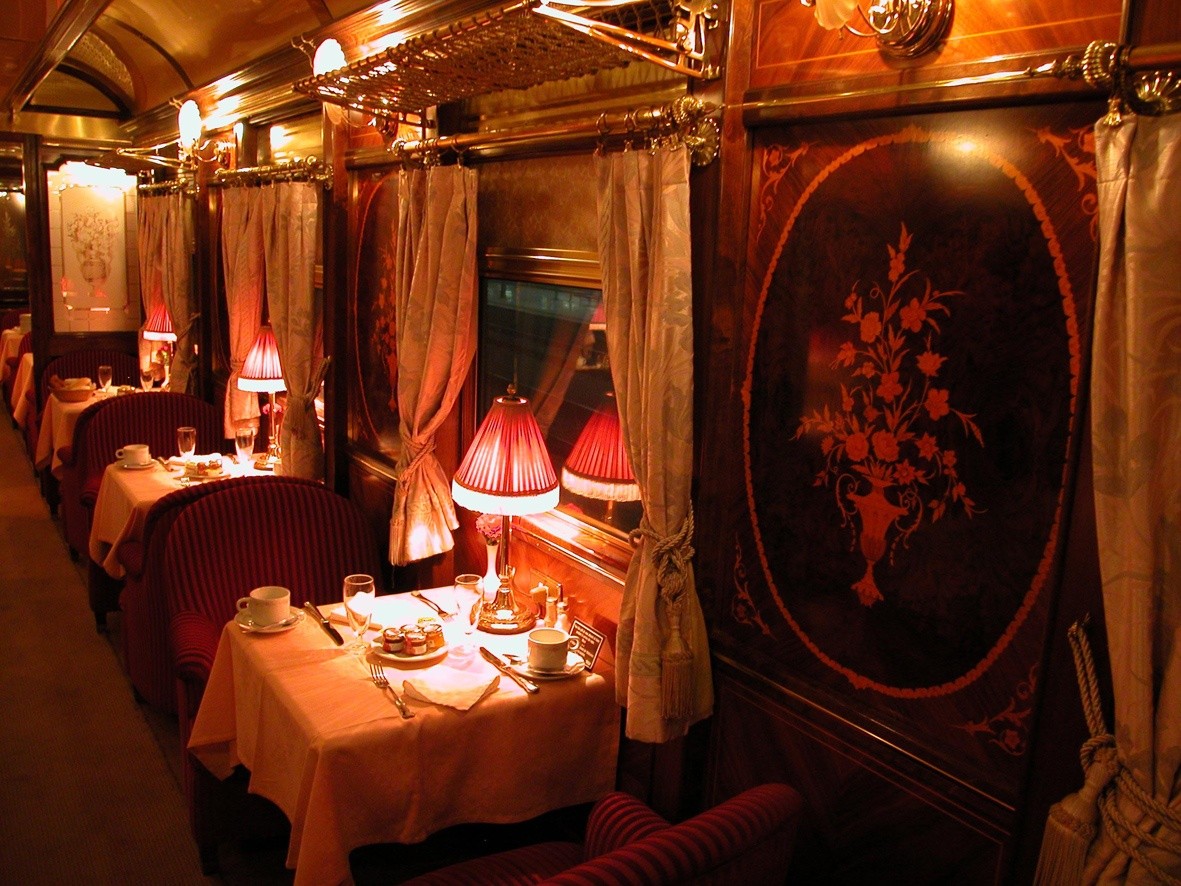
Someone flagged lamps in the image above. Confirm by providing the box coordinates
[313,38,399,137]
[144,303,178,391]
[178,100,230,170]
[238,326,288,470]
[562,392,641,526]
[451,381,559,635]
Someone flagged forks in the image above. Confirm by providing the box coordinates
[369,660,416,718]
[227,453,240,464]
[411,590,454,620]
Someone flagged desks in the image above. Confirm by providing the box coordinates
[88,455,281,633]
[225,583,605,886]
[9,353,41,457]
[0,328,36,382]
[38,384,143,520]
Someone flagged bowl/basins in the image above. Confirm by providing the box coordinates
[49,387,96,402]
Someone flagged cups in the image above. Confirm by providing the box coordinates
[236,586,291,626]
[528,628,580,671]
[235,428,254,465]
[140,372,153,392]
[177,427,196,457]
[116,444,149,465]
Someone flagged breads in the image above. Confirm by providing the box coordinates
[49,375,92,389]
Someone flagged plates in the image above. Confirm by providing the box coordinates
[117,459,158,469]
[370,630,448,663]
[185,470,230,479]
[510,651,585,681]
[234,606,306,633]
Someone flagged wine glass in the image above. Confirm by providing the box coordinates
[98,366,112,399]
[454,574,484,656]
[342,574,375,655]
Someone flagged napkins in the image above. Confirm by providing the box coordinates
[403,664,501,715]
[329,591,418,634]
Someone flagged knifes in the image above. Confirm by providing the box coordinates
[479,646,540,694]
[305,600,344,646]
[158,456,173,471]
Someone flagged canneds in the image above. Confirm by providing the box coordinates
[381,624,445,657]
[184,459,223,477]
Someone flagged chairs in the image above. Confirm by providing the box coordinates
[33,349,802,886]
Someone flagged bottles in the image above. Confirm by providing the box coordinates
[544,597,572,633]
